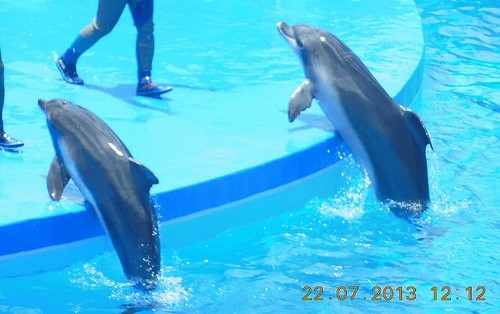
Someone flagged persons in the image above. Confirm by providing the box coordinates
[55,0,173,97]
[0,49,26,149]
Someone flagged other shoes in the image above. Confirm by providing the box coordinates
[137,72,173,94]
[56,50,83,84]
[0,132,24,149]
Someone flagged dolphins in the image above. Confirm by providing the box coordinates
[276,21,435,219]
[37,98,161,314]
